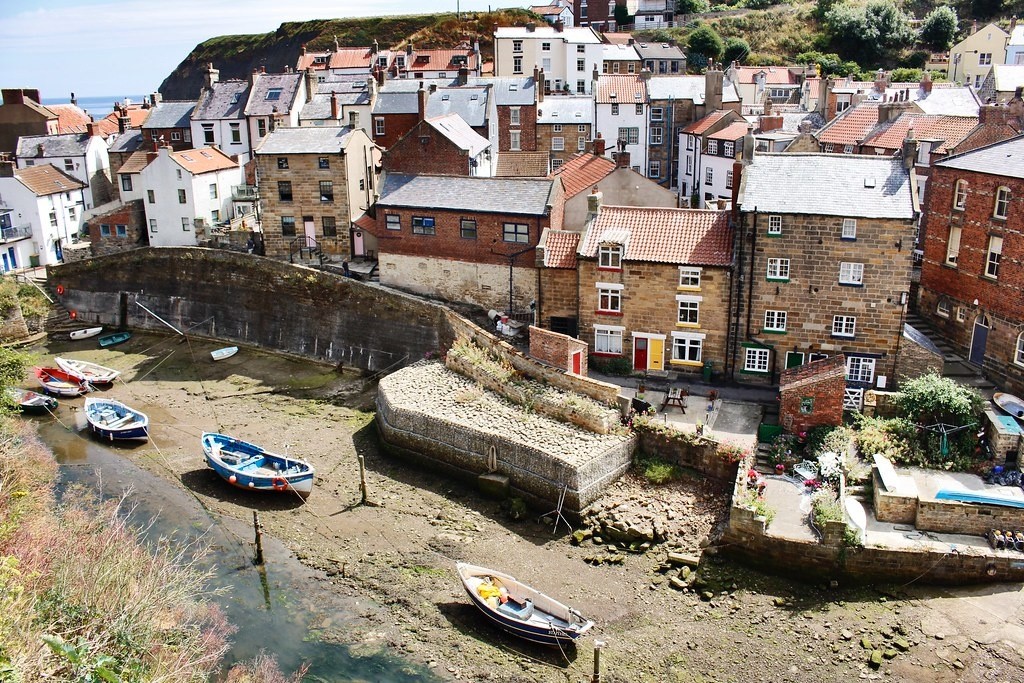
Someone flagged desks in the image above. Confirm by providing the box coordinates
[660,388,687,414]
[499,597,534,620]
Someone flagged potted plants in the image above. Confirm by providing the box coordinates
[648,406,657,416]
[635,369,648,393]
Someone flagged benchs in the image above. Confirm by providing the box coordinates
[793,459,819,488]
[23,395,41,404]
[681,396,687,408]
[75,364,86,372]
[108,413,134,427]
[661,391,668,405]
[232,454,267,470]
[112,336,117,342]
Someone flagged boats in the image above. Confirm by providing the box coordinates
[70,326,102,341]
[456,562,595,649]
[210,346,239,361]
[993,392,1024,422]
[200,430,316,499]
[98,331,130,348]
[83,397,150,441]
[33,366,89,397]
[54,356,120,384]
[5,387,58,415]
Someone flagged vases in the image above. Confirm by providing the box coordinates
[983,472,989,479]
[709,396,715,401]
[806,486,812,492]
[775,468,784,475]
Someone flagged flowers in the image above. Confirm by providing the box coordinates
[803,479,821,490]
[975,460,995,472]
[747,469,761,481]
[708,389,717,396]
[768,434,791,470]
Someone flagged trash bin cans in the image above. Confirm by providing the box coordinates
[704,364,712,377]
[30,254,40,267]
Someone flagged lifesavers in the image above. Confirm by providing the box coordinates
[272,476,289,491]
[25,391,36,401]
[56,285,64,294]
[70,310,77,319]
